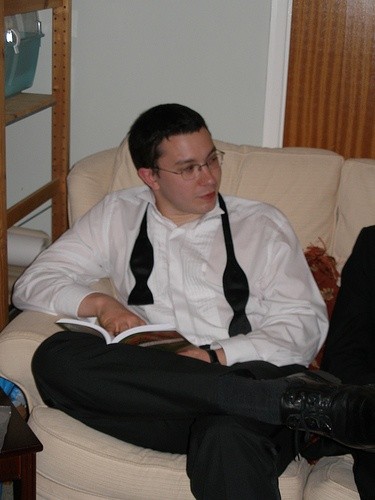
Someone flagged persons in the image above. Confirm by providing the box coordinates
[10,103,375,500]
[307,224,375,500]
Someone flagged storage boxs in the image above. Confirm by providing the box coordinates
[4,11,45,98]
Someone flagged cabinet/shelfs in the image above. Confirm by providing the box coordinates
[0,0,71,331]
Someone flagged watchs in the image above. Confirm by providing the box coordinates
[199,344,220,365]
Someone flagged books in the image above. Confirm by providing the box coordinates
[55,317,192,356]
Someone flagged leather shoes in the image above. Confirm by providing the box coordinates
[282,369,375,450]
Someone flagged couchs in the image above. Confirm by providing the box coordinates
[0,136,375,500]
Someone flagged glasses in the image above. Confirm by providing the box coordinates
[150,148,225,181]
